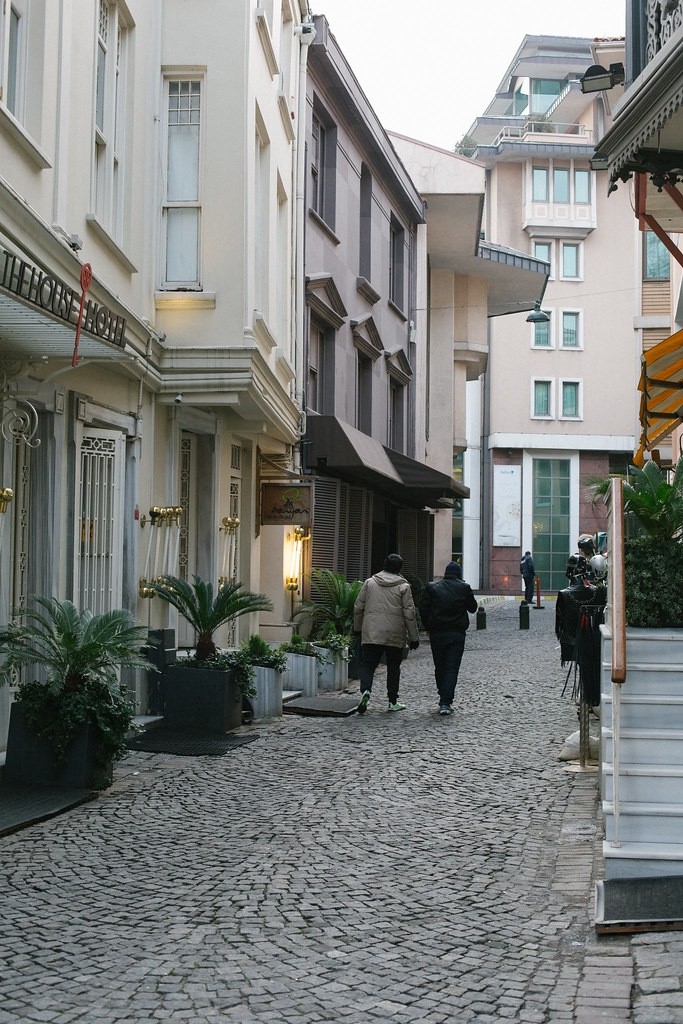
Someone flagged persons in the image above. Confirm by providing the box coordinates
[518,550,537,604]
[353,553,420,715]
[420,562,478,716]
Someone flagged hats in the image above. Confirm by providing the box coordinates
[445,561,461,575]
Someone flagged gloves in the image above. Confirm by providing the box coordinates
[410,641,419,650]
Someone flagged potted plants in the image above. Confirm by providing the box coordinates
[308,629,352,691]
[145,572,274,731]
[240,633,289,718]
[0,591,167,787]
[278,634,319,696]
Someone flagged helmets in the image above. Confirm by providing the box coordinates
[566,553,588,579]
[590,554,608,578]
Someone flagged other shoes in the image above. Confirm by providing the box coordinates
[388,702,406,711]
[527,601,535,604]
[357,690,370,714]
[439,705,452,714]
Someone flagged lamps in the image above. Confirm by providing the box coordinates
[139,505,183,600]
[285,525,305,590]
[0,487,14,513]
[525,298,550,323]
[578,62,625,94]
[218,516,239,590]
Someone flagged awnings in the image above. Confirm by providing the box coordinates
[303,414,471,508]
